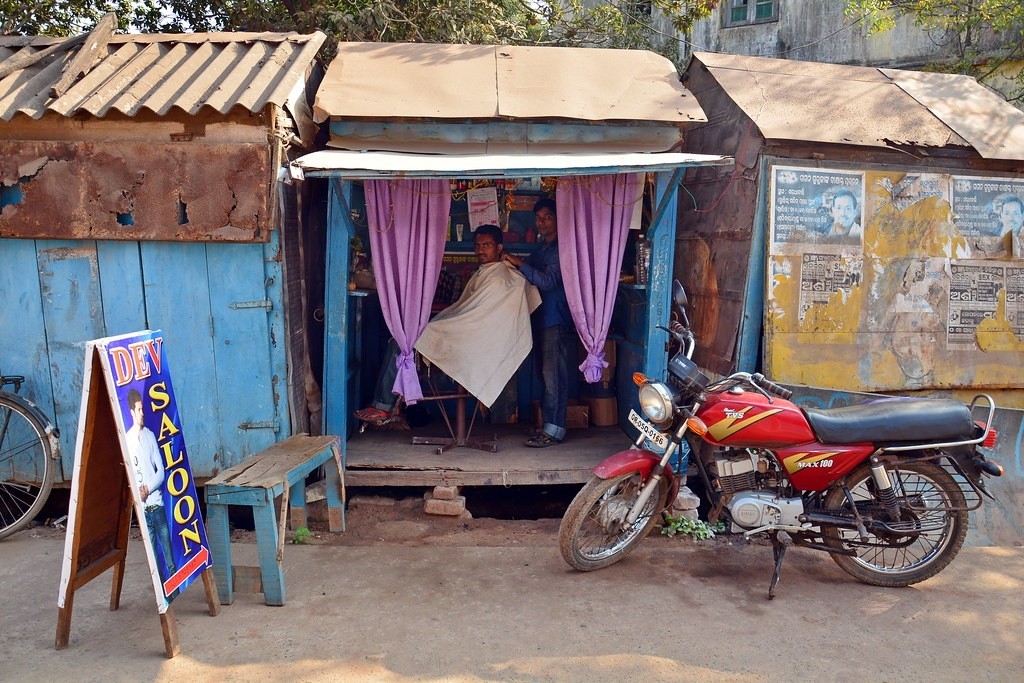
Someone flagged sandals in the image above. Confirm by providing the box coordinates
[524,433,559,447]
[522,425,543,436]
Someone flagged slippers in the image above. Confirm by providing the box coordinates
[353,407,401,422]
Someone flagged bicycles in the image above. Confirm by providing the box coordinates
[0,375,60,542]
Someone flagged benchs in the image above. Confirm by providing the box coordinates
[204,433,346,605]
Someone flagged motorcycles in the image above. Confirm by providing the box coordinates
[558,278,1005,601]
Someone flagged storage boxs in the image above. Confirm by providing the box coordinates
[536,399,589,427]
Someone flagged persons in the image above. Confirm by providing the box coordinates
[825,194,863,245]
[499,197,575,448]
[997,196,1024,261]
[351,223,526,423]
[121,389,181,602]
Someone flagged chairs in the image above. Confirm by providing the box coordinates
[359,279,526,455]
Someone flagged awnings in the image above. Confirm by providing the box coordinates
[279,131,739,201]
